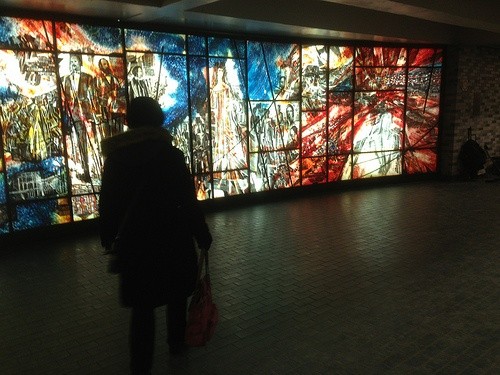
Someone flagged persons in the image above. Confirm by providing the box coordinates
[97,97,213,375]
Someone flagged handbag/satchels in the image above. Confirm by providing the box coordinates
[184,247,218,346]
